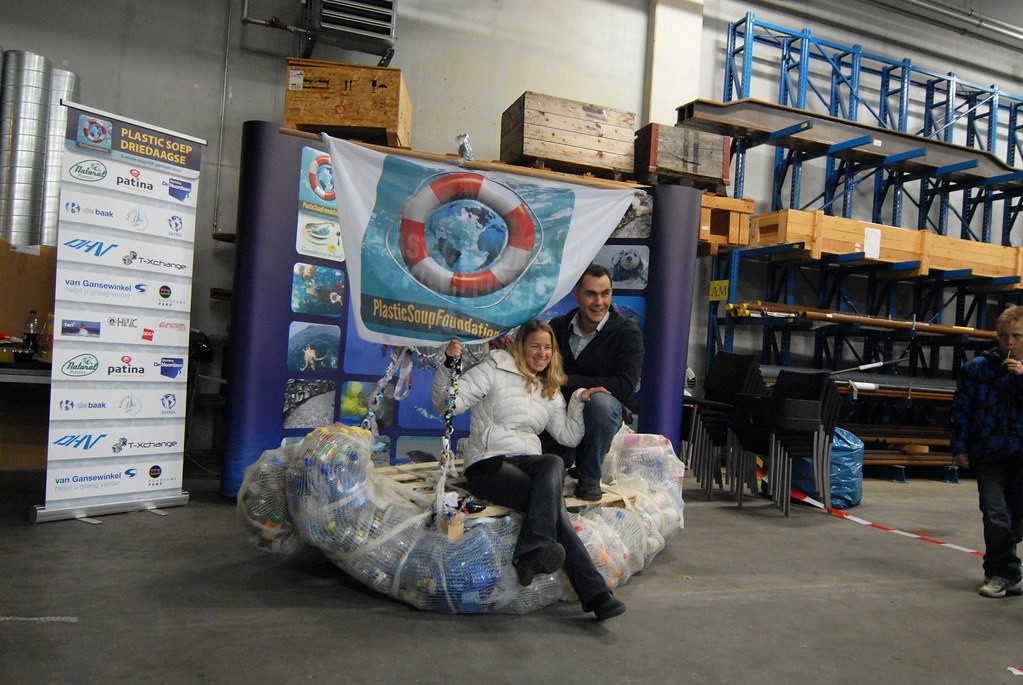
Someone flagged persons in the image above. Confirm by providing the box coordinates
[548,263,645,501]
[432,319,626,620]
[948,304,1023,598]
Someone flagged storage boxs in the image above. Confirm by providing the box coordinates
[284,57,412,148]
[495,89,636,176]
[631,122,733,197]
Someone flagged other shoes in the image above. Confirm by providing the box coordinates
[569,465,581,480]
[576,479,602,499]
[594,591,624,621]
[516,542,565,586]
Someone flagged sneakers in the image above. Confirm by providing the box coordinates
[979,575,1023,598]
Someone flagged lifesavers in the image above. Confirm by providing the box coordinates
[400,171,537,297]
[310,156,337,201]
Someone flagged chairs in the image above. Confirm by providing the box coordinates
[677,352,842,518]
